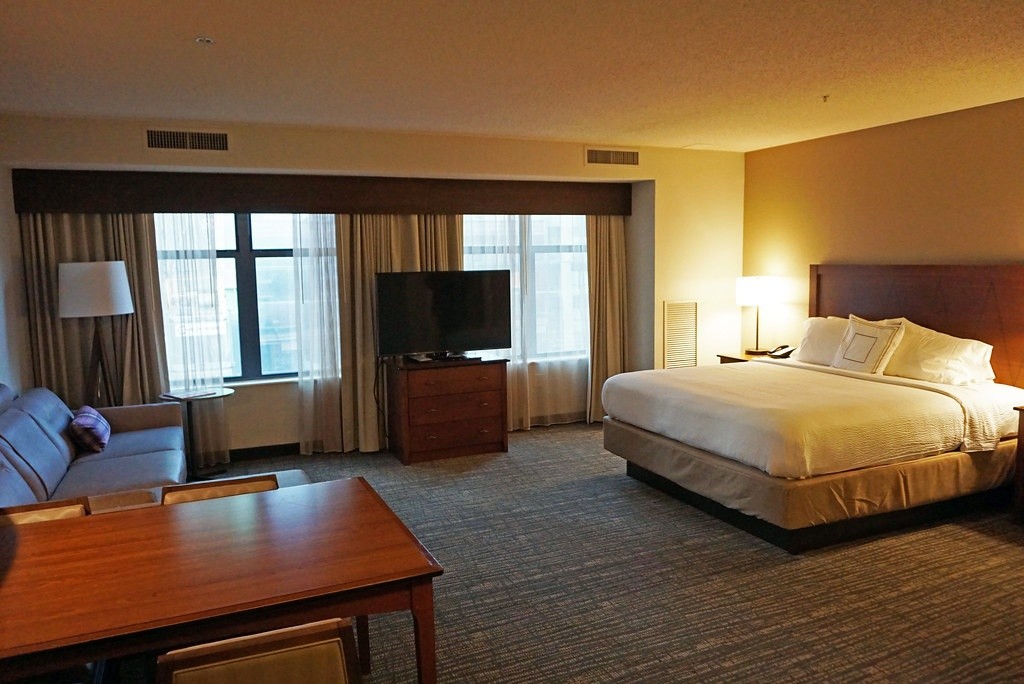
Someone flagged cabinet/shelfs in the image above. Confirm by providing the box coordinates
[383,353,511,466]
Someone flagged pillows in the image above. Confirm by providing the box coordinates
[71,405,111,453]
[830,313,905,376]
[883,317,998,386]
[793,315,902,366]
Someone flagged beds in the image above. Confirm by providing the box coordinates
[600,265,1024,556]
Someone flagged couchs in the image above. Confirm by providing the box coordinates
[0,383,189,509]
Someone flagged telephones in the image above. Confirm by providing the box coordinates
[767,344,797,358]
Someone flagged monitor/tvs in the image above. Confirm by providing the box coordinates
[375,270,511,363]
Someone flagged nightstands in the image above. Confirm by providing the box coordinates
[716,352,769,365]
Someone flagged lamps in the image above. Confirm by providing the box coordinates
[58,260,137,407]
[735,275,769,356]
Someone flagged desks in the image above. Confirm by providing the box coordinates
[157,387,236,477]
[0,474,443,684]
